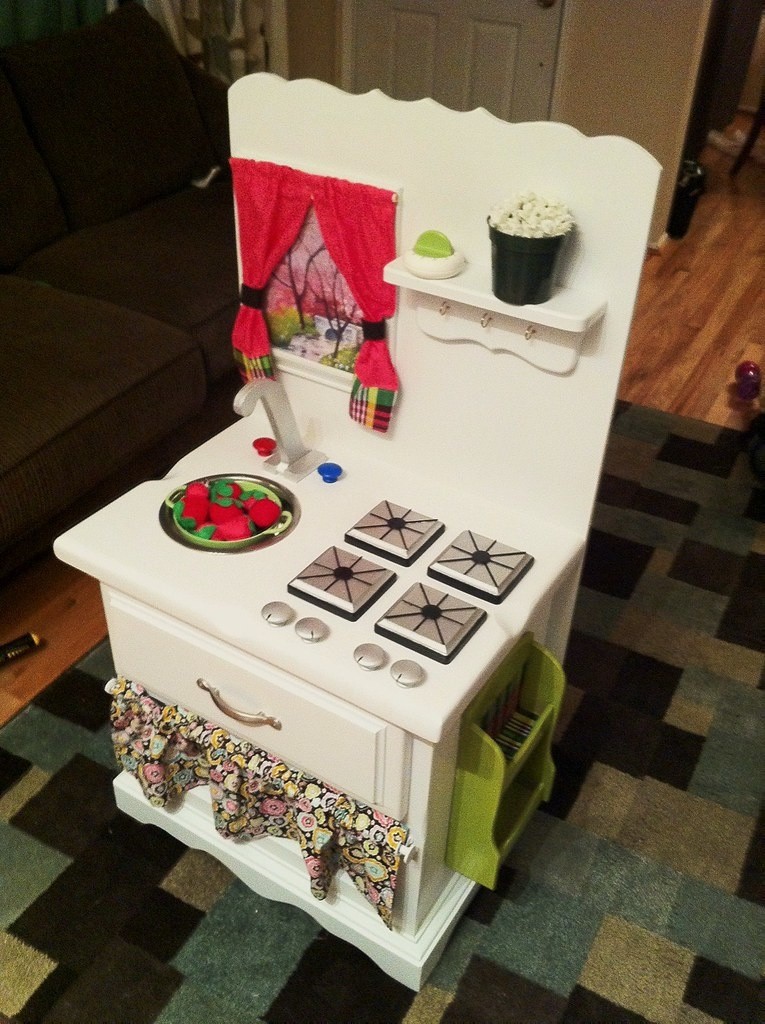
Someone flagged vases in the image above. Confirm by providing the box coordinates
[487,213,573,307]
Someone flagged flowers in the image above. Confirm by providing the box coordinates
[491,192,572,238]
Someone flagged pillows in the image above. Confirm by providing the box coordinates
[11,0,209,231]
[0,52,68,277]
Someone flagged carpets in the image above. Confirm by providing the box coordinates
[1,398,765,1024]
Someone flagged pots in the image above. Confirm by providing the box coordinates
[165,480,292,549]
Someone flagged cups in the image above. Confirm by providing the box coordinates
[487,213,566,306]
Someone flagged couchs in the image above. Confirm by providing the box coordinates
[0,0,242,583]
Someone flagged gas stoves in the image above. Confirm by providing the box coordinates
[261,500,534,687]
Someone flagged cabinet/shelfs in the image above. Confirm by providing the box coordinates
[92,572,578,992]
[444,631,564,891]
